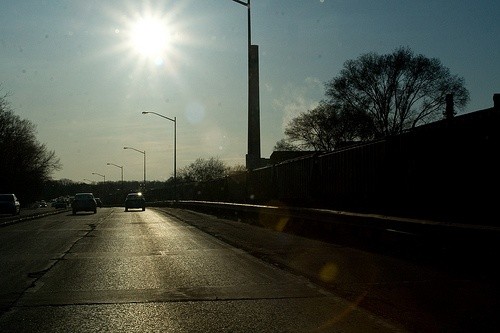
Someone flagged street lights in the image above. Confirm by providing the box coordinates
[91,172,105,206]
[83,178,94,185]
[142,111,178,204]
[106,162,123,205]
[123,146,146,195]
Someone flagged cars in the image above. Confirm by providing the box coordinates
[95,198,103,208]
[50,194,76,210]
[71,193,98,215]
[124,192,146,211]
[0,193,21,216]
[40,201,48,208]
